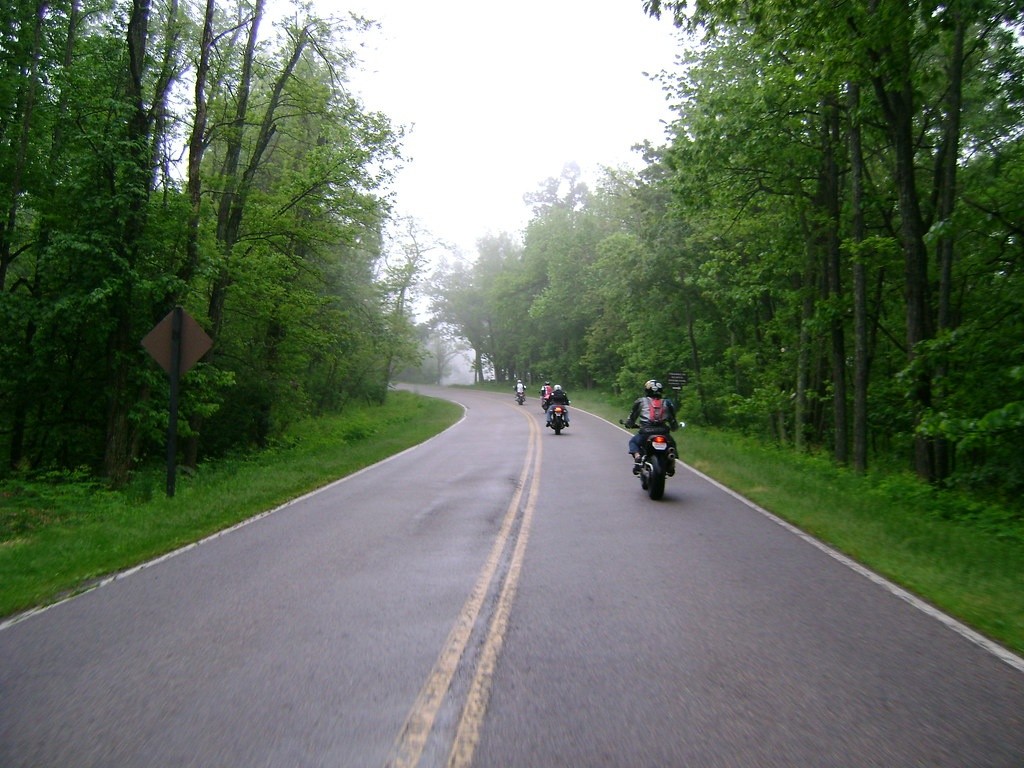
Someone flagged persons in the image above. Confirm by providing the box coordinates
[541,381,554,400]
[545,384,570,427]
[626,379,679,476]
[513,379,527,401]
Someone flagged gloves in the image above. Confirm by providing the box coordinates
[625,422,634,429]
[671,425,678,432]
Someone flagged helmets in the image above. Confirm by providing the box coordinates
[517,379,521,383]
[545,381,550,385]
[644,379,663,396]
[553,385,562,391]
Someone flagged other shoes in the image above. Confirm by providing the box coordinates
[546,422,550,427]
[565,421,569,427]
[668,467,675,477]
[633,456,641,475]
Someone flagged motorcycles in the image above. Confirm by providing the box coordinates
[514,388,526,405]
[547,400,572,435]
[619,419,685,501]
[539,392,550,413]
